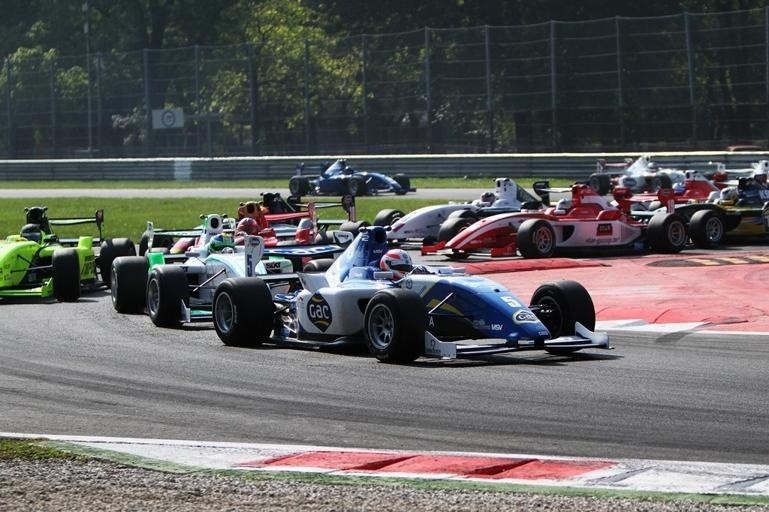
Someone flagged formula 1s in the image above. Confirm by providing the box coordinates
[289,158,417,197]
[212,225,616,360]
[1,206,138,303]
[109,155,769,329]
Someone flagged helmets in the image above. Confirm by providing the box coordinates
[554,198,573,214]
[343,165,354,174]
[237,217,257,235]
[380,247,413,280]
[207,232,236,254]
[719,187,737,204]
[480,192,496,206]
[20,223,42,245]
[672,182,686,194]
